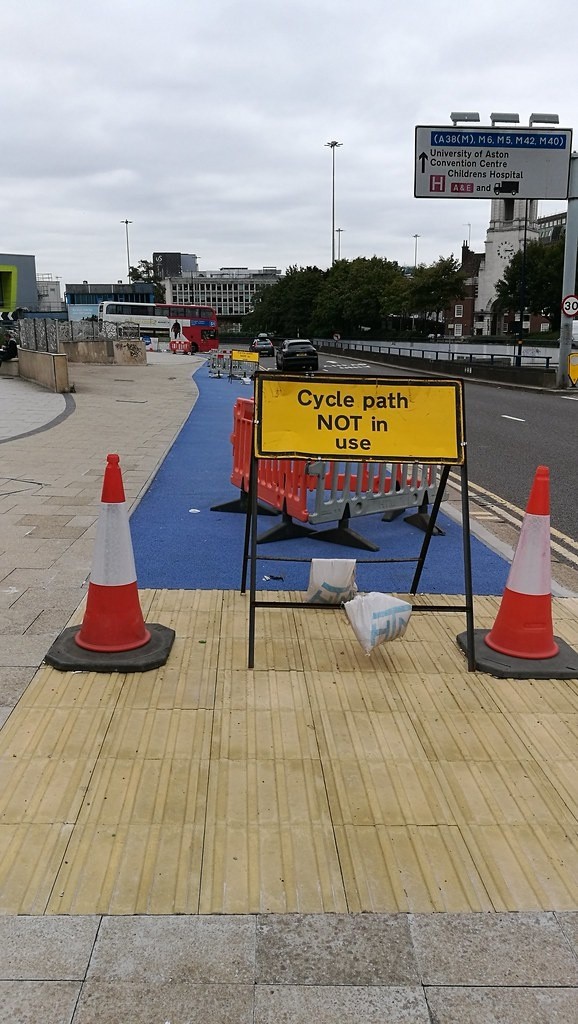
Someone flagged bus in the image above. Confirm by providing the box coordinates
[98,301,221,354]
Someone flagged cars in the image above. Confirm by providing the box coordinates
[249,334,275,357]
[275,338,319,372]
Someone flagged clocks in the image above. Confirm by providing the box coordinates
[496,241,514,260]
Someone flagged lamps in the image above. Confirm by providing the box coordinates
[480,309,484,311]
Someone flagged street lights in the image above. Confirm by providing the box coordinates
[120,219,134,284]
[323,140,344,268]
[412,233,420,267]
[335,227,345,260]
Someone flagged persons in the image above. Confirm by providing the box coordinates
[171,319,180,339]
[0,333,18,367]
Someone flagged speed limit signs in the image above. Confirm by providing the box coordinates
[561,295,578,317]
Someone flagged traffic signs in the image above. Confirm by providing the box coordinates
[412,124,573,201]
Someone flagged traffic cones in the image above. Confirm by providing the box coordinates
[452,464,577,680]
[43,449,177,674]
